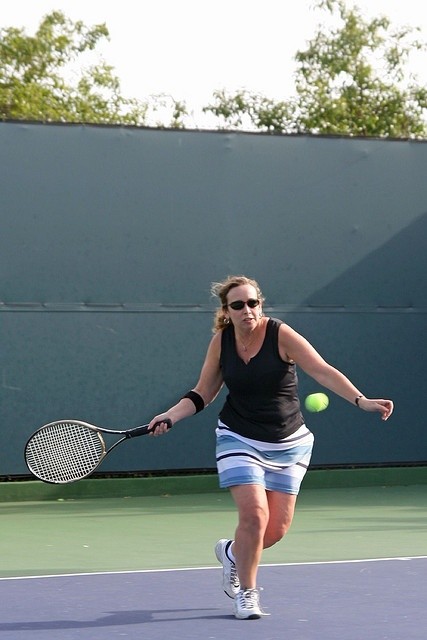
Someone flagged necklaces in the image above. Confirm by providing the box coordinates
[237,332,255,353]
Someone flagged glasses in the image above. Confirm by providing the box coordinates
[226,299,258,309]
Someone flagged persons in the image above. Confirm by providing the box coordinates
[148,276,395,618]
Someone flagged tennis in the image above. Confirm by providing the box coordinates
[303,393,328,412]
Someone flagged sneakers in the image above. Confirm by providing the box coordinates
[215,538,239,598]
[233,587,271,619]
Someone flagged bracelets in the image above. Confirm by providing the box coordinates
[355,394,364,407]
